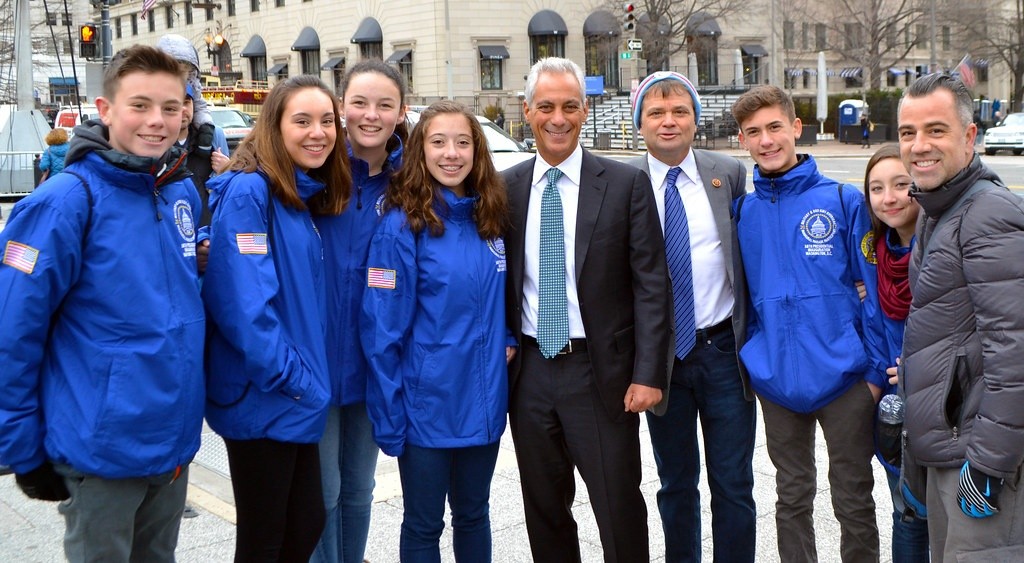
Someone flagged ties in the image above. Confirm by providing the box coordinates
[665,167,697,360]
[538,168,570,360]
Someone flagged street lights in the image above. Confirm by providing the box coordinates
[204,33,225,66]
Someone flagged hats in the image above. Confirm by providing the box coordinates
[633,71,701,129]
[185,84,194,100]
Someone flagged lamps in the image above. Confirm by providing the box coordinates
[205,20,223,70]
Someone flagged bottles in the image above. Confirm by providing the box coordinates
[878,394,905,425]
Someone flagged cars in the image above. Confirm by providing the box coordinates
[402,103,537,173]
[982,113,1024,157]
[205,103,257,150]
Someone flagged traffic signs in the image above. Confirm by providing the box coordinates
[627,39,643,52]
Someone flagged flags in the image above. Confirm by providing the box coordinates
[952,55,975,89]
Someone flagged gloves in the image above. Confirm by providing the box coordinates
[956,461,1001,518]
[15,461,70,501]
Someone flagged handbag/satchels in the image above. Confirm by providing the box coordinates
[39,169,51,184]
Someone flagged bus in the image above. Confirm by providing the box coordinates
[200,74,276,121]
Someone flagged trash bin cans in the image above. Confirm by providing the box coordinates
[508,120,523,142]
[596,131,612,150]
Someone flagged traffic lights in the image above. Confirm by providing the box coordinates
[623,2,634,31]
[76,24,97,58]
[95,27,103,59]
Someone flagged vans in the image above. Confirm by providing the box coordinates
[54,101,100,143]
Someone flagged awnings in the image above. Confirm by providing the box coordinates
[321,57,344,70]
[527,10,568,36]
[741,44,769,58]
[635,11,671,40]
[583,10,621,37]
[479,45,510,60]
[291,27,319,51]
[267,63,287,74]
[385,50,413,63]
[350,17,381,44]
[787,66,863,79]
[685,11,722,39]
[888,66,919,77]
[240,34,266,57]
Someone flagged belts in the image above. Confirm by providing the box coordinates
[696,319,731,340]
[521,335,585,356]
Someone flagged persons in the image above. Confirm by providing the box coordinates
[854,144,932,563]
[201,77,355,562]
[497,58,674,563]
[896,72,1023,563]
[1,34,232,563]
[356,103,517,562]
[861,114,871,149]
[306,63,415,563]
[731,85,879,562]
[620,72,756,563]
